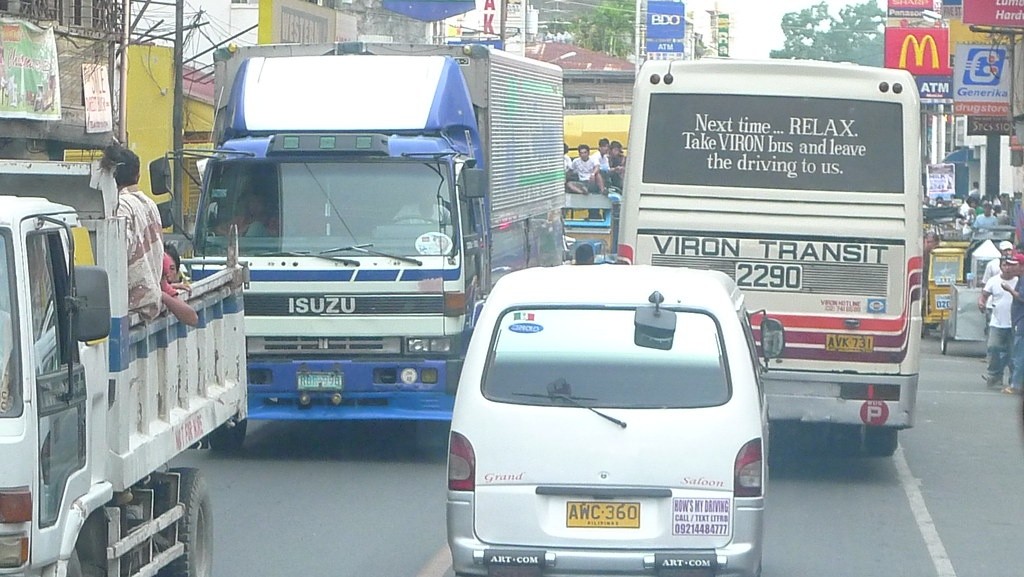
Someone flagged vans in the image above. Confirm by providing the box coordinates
[442,245,785,577]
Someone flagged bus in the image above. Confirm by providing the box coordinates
[549,52,930,461]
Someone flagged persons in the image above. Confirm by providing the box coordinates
[564,139,626,195]
[214,195,279,237]
[575,245,594,265]
[923,182,1024,234]
[100,146,199,326]
[978,241,1024,394]
[392,188,450,225]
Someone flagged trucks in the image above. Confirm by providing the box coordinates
[150,40,568,457]
[0,158,255,577]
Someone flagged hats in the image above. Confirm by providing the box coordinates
[999,241,1013,251]
[1006,254,1024,265]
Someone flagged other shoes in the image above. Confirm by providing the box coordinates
[983,374,989,381]
[1001,386,1024,395]
